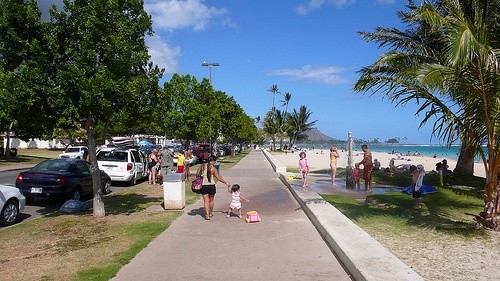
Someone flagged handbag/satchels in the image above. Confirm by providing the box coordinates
[191,177,203,192]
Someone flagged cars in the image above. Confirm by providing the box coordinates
[96,141,244,186]
[0,184,26,226]
[15,158,111,202]
[59,146,88,161]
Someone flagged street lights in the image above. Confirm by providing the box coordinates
[202,63,220,156]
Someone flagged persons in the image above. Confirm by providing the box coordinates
[174,147,192,180]
[434,154,437,158]
[293,144,324,154]
[357,144,374,193]
[441,159,449,170]
[392,149,418,160]
[330,146,340,185]
[299,152,310,188]
[353,163,361,190]
[373,158,380,170]
[410,165,422,209]
[147,143,163,185]
[196,155,231,220]
[226,184,250,219]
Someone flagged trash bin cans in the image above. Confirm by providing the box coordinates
[162,173,185,211]
